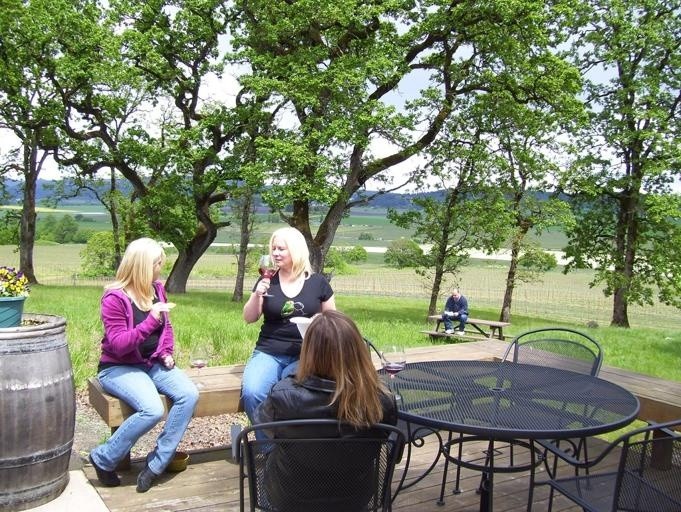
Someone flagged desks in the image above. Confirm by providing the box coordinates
[373,361,642,512]
[427,315,511,342]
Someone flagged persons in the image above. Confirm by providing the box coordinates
[241,224,335,456]
[251,308,399,512]
[89,237,200,490]
[442,287,469,335]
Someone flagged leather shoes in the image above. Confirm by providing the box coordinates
[88,454,120,487]
[137,455,157,492]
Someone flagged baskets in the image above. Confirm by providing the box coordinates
[166,452,189,472]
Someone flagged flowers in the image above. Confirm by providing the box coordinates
[0,266,30,297]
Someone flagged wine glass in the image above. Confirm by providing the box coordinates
[379,343,408,401]
[189,345,209,388]
[257,255,277,297]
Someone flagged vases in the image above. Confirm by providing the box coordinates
[0,297,29,328]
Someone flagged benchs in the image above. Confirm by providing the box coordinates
[419,330,491,342]
[442,327,517,339]
[88,344,681,471]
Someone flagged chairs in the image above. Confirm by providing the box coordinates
[230,419,404,512]
[527,420,681,512]
[362,336,464,498]
[478,326,602,493]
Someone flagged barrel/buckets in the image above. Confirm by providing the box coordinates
[0,312,76,512]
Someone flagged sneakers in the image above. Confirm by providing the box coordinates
[444,329,455,334]
[456,331,464,335]
